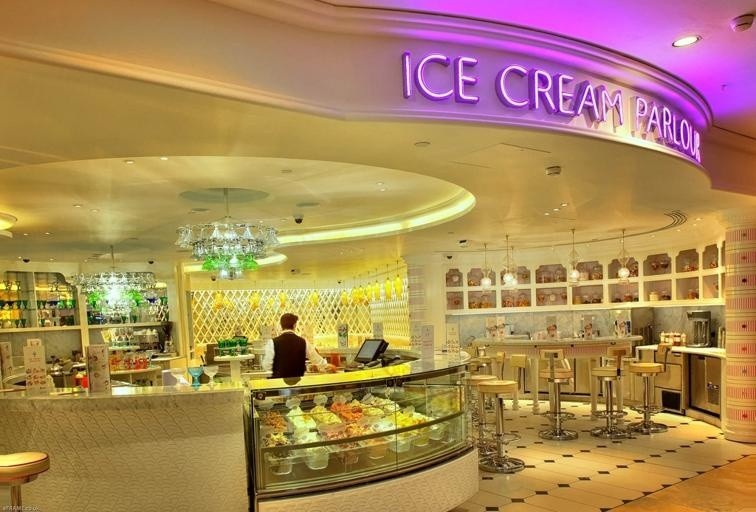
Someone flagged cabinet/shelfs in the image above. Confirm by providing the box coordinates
[248,349,478,512]
[0,271,80,333]
[445,241,726,315]
[80,364,162,387]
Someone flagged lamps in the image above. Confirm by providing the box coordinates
[570,229,580,280]
[214,259,402,310]
[545,166,561,176]
[174,188,281,280]
[70,246,158,319]
[617,228,630,278]
[479,235,518,290]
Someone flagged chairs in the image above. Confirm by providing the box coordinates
[463,340,675,473]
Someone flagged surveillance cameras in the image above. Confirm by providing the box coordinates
[211,276,216,281]
[148,260,154,264]
[23,258,30,262]
[447,256,452,259]
[294,215,303,224]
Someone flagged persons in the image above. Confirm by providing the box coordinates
[497,322,505,335]
[488,324,497,336]
[584,323,593,337]
[260,312,338,379]
[546,323,557,339]
[614,320,630,335]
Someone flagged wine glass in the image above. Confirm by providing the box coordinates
[0,318,27,328]
[170,368,186,388]
[188,367,204,387]
[203,366,218,386]
[40,318,46,327]
[120,313,139,323]
[37,298,76,310]
[660,260,669,274]
[100,327,134,348]
[0,300,28,310]
[146,350,155,367]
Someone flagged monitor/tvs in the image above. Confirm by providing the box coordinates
[354,339,388,362]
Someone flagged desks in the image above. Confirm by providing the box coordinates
[213,354,256,380]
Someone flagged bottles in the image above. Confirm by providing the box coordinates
[660,331,687,346]
[687,288,699,299]
[649,289,671,301]
[613,291,638,302]
[573,292,601,304]
[537,291,567,306]
[110,349,147,370]
[578,265,603,281]
[537,268,565,283]
[469,295,491,308]
[502,293,529,307]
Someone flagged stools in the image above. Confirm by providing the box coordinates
[0,451,50,512]
